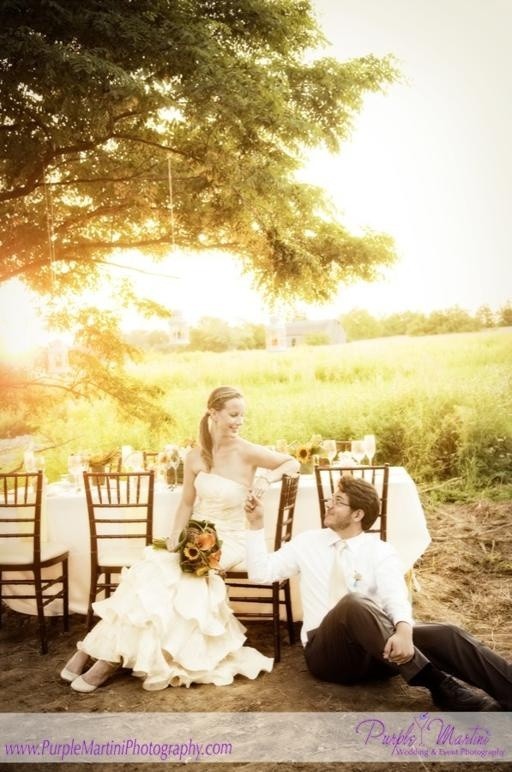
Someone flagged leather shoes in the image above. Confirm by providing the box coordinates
[59,652,89,682]
[70,676,96,693]
[432,676,487,709]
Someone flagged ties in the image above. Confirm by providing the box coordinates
[329,541,347,603]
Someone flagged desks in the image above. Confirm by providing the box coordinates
[2,465,407,624]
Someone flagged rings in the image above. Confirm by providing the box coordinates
[257,494,262,498]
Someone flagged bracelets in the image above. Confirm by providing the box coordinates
[260,476,271,486]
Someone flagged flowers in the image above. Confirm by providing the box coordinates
[291,444,327,465]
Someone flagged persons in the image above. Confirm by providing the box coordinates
[244,476,512,712]
[61,385,303,694]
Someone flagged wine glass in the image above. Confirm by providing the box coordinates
[23,444,143,491]
[320,435,376,469]
[157,447,181,492]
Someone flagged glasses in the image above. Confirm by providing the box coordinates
[328,493,351,508]
